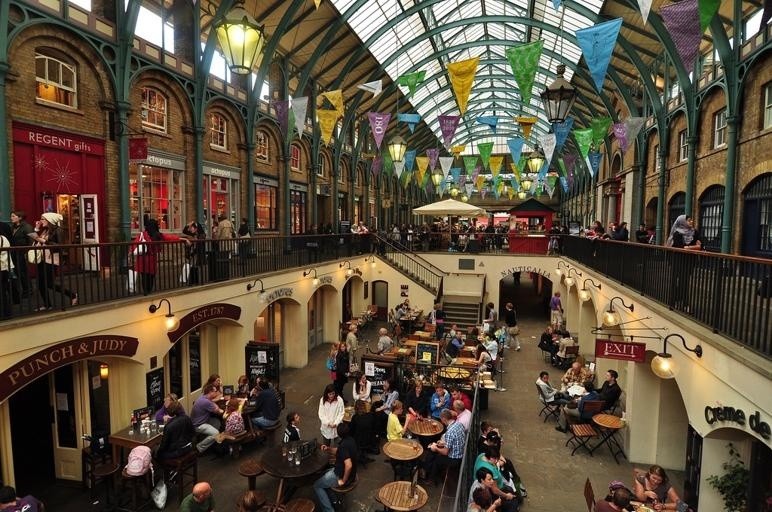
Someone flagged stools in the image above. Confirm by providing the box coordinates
[329,472,359,512]
[286,498,316,512]
[238,456,266,490]
[235,491,266,510]
[329,452,337,466]
[542,350,551,364]
[565,424,598,457]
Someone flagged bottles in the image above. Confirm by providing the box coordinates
[407,406,420,420]
[377,380,386,392]
[311,438,318,456]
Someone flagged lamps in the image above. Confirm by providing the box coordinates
[213,0,269,76]
[149,255,377,333]
[100,365,109,379]
[554,261,702,380]
[387,0,578,203]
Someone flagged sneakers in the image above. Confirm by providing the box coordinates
[505,344,509,348]
[515,347,520,351]
[555,426,566,432]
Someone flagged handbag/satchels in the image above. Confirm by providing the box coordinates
[127,446,152,476]
[507,325,520,336]
[326,357,337,371]
[151,480,168,510]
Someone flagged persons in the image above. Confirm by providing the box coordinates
[512,272,522,286]
[590,215,702,310]
[1,213,252,320]
[2,487,44,512]
[537,292,622,435]
[305,216,570,265]
[284,300,526,511]
[116,374,283,511]
[596,465,680,512]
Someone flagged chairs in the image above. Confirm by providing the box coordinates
[190,396,286,461]
[632,468,647,495]
[584,477,596,512]
[535,372,627,421]
[81,435,119,499]
[166,429,205,503]
[343,397,355,406]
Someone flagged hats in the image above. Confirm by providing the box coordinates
[487,431,500,442]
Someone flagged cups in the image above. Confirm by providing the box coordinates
[567,382,580,394]
[412,440,418,452]
[220,397,225,406]
[164,412,167,422]
[613,226,615,232]
[414,489,419,502]
[282,439,303,465]
[130,406,157,436]
[652,497,658,509]
[556,341,559,343]
[428,411,437,432]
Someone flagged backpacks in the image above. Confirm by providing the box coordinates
[224,411,245,436]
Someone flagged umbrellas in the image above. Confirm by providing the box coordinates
[411,198,486,215]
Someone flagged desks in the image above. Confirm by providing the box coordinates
[590,412,628,465]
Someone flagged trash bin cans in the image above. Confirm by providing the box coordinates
[205,249,232,282]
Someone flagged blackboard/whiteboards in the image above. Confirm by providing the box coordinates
[415,341,439,379]
